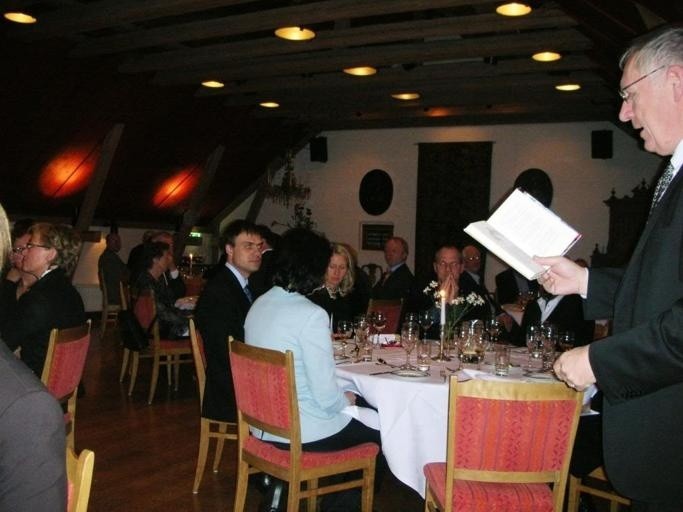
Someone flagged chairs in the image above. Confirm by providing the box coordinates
[97,262,198,406]
[488,270,519,316]
[229,335,379,512]
[63,446,95,512]
[190,319,237,493]
[39,318,92,456]
[423,374,583,512]
[366,298,404,333]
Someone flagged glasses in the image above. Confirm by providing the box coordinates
[12,247,24,254]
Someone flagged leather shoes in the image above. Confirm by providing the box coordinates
[249,468,274,491]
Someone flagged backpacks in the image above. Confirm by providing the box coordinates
[116,308,150,351]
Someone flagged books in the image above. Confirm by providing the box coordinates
[461,183,582,279]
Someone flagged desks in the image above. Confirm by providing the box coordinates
[334,340,598,501]
[502,303,523,327]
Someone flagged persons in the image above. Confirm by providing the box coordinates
[528,18,682,510]
[1,217,195,412]
[0,203,69,512]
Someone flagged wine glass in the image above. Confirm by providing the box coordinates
[516,288,538,307]
[336,310,577,378]
[178,266,204,282]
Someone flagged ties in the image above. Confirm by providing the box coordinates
[244,286,253,306]
[646,163,676,222]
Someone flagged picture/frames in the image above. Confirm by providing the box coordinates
[512,165,557,212]
[353,165,396,221]
[358,224,396,254]
